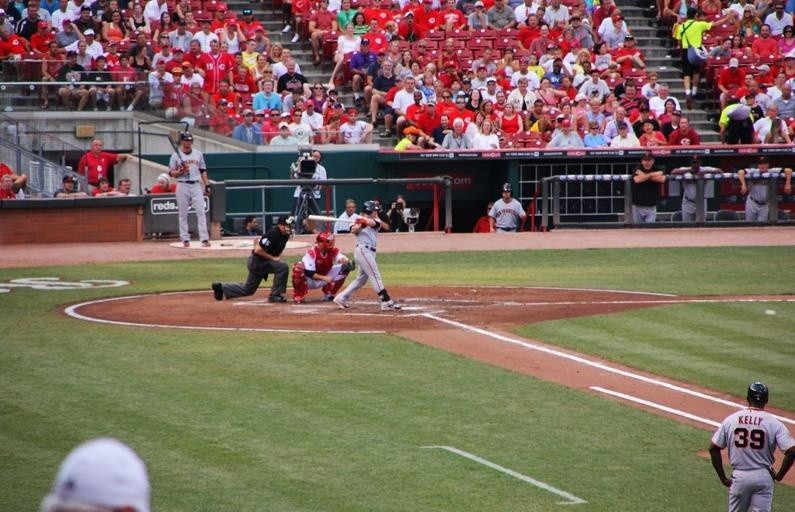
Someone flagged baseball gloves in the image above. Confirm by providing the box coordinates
[339,260,355,274]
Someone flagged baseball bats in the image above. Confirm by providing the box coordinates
[308,215,355,222]
[167,133,190,176]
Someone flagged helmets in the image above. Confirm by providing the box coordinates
[503,183,512,192]
[362,201,381,211]
[316,232,335,248]
[181,131,193,140]
[747,382,768,404]
[278,215,296,235]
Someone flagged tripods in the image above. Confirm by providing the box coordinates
[293,192,328,233]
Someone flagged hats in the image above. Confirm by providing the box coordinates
[348,107,358,116]
[306,100,314,109]
[618,122,628,130]
[575,93,586,103]
[643,151,652,160]
[228,18,236,28]
[156,50,191,76]
[243,9,252,17]
[361,38,369,44]
[471,0,490,13]
[611,14,624,26]
[243,108,291,131]
[404,10,415,18]
[672,109,682,116]
[690,156,701,163]
[476,64,512,86]
[757,156,768,164]
[561,118,572,128]
[624,34,636,43]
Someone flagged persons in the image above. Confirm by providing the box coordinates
[332,200,402,311]
[709,381,795,512]
[212,213,297,302]
[292,233,354,304]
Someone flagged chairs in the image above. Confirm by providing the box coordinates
[1,2,793,151]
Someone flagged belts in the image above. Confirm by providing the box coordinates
[180,180,199,184]
[366,245,376,252]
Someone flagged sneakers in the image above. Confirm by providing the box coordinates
[212,283,223,300]
[381,300,401,311]
[268,294,287,303]
[323,294,349,309]
